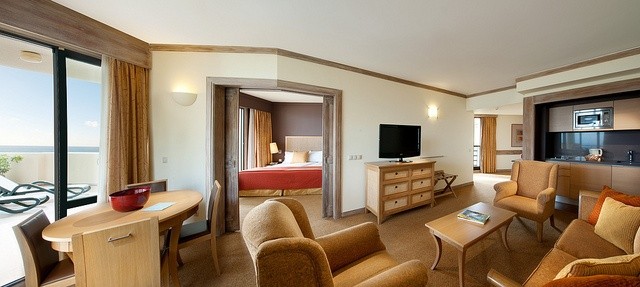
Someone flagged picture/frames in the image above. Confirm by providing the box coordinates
[511,124,523,147]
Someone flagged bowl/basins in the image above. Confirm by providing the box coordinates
[108,186,152,213]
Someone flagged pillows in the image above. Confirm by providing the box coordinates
[593,197,640,255]
[292,151,308,163]
[553,254,640,280]
[587,184,640,225]
[285,151,293,162]
[543,274,640,287]
[307,150,322,162]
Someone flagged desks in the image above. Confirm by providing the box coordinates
[433,170,458,205]
[41,189,204,287]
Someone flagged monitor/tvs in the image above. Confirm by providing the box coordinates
[379,123,421,163]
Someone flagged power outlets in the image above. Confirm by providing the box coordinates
[278,149,282,154]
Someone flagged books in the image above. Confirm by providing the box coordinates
[143,202,176,211]
[457,209,490,225]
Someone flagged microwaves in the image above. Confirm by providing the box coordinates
[574,107,615,131]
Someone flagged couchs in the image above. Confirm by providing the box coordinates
[494,159,559,243]
[486,190,639,287]
[241,197,428,287]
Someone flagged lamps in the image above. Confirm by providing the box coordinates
[270,143,279,165]
[170,92,197,105]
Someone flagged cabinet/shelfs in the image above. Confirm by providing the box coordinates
[549,105,573,132]
[364,158,436,225]
[570,164,640,200]
[474,146,481,170]
[555,164,569,198]
[613,98,640,130]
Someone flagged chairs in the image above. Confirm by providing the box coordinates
[0,195,49,213]
[125,179,169,238]
[12,209,75,287]
[71,216,171,286]
[162,179,222,276]
[0,173,91,198]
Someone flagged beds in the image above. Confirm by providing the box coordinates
[239,136,323,197]
